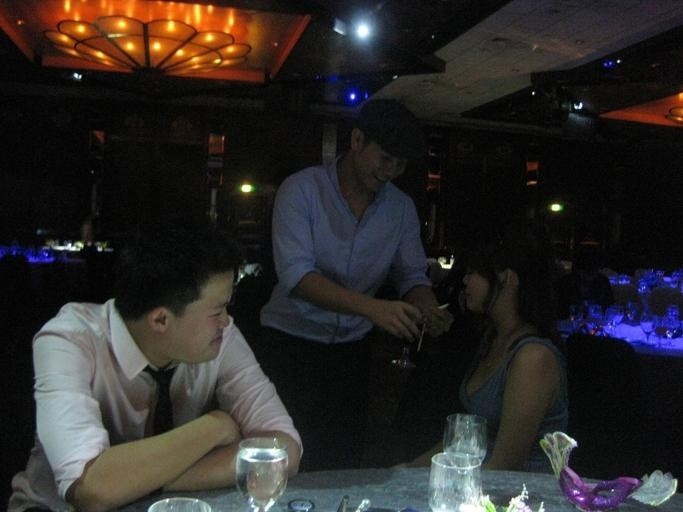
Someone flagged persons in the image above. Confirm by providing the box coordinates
[251,96,456,471]
[456,212,570,472]
[7,221,304,511]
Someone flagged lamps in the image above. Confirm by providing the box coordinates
[42,15,251,80]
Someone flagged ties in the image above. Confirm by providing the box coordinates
[144,366,176,436]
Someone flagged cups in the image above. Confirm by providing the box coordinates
[428,413,488,511]
[148,438,288,511]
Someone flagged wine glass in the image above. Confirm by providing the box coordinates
[391,316,425,370]
[556,306,681,349]
